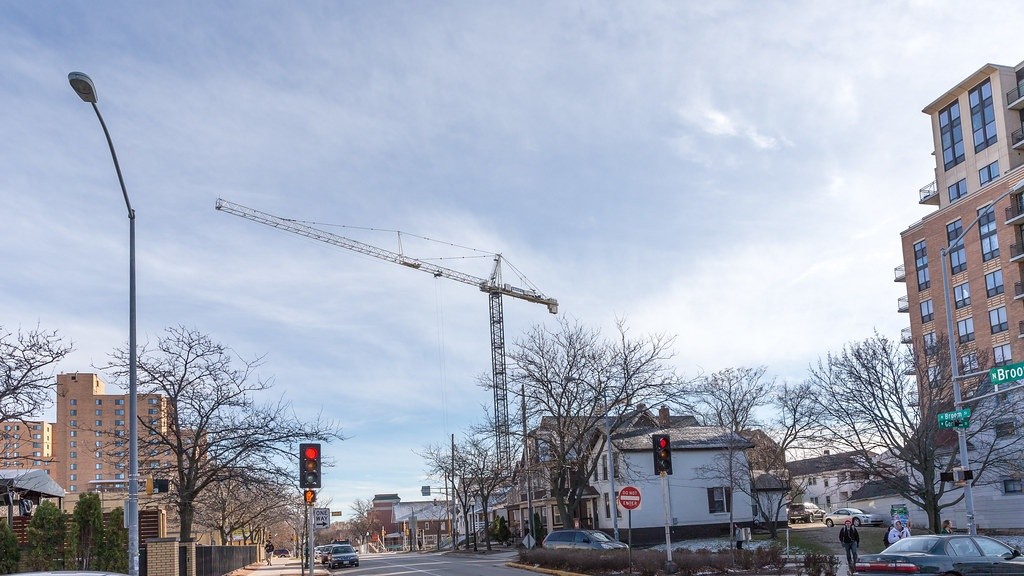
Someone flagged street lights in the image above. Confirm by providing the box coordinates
[938,178,1024,537]
[62,70,139,576]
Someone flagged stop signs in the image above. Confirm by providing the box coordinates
[618,487,643,509]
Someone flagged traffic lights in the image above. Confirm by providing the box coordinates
[155,478,169,493]
[651,433,674,477]
[940,471,954,483]
[305,490,317,504]
[964,469,974,481]
[299,443,321,490]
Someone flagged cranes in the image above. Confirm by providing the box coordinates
[212,197,560,481]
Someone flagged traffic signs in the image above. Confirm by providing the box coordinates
[313,507,331,529]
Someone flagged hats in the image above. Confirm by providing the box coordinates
[845,520,851,525]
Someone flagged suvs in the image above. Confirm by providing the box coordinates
[787,502,827,524]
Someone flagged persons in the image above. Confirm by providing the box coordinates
[265,540,274,566]
[839,520,859,575]
[883,521,911,549]
[941,520,953,535]
[734,523,743,549]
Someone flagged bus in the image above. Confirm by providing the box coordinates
[330,538,351,546]
[542,529,630,550]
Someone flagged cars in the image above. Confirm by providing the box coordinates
[314,545,326,560]
[851,534,1024,576]
[327,545,359,568]
[319,543,341,565]
[273,549,292,559]
[822,507,884,527]
